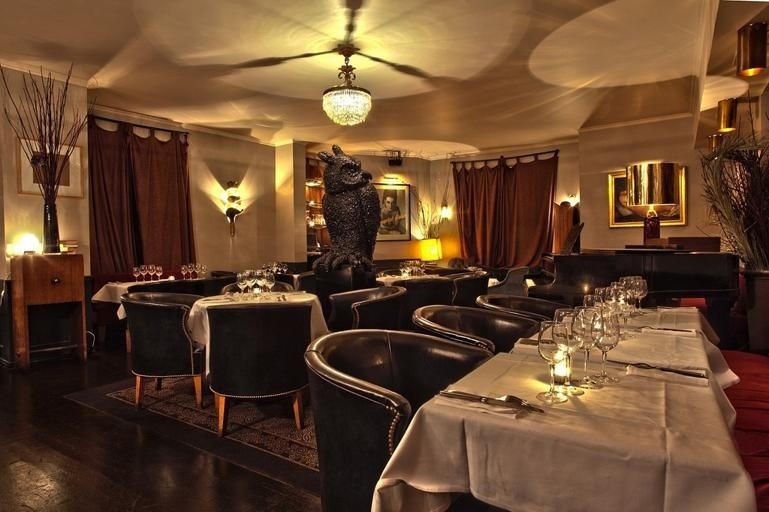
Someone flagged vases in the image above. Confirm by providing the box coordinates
[41,199,62,254]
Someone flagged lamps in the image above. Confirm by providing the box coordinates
[717,99,736,134]
[736,21,767,75]
[322,43,372,125]
[622,157,679,245]
[222,179,243,237]
[411,187,453,262]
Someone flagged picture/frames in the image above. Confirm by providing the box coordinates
[15,136,86,200]
[607,161,687,229]
[368,183,412,241]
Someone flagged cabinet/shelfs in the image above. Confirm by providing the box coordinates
[11,254,86,367]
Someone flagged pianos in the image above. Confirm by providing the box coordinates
[523,245,738,350]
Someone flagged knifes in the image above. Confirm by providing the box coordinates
[439,390,544,413]
[606,359,704,378]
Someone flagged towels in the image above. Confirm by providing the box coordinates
[627,364,711,387]
[642,324,698,338]
[657,305,699,314]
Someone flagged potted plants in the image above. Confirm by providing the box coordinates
[699,127,768,353]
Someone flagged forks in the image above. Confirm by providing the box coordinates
[448,389,533,413]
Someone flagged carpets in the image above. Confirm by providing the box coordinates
[63,364,325,473]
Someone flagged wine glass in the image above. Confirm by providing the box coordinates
[552,308,585,396]
[262,256,288,275]
[620,306,647,343]
[200,265,208,279]
[536,320,568,404]
[399,259,426,279]
[155,265,163,282]
[237,271,248,301]
[194,263,201,280]
[583,276,648,306]
[132,267,140,283]
[147,264,156,283]
[591,309,619,385]
[570,305,604,389]
[181,265,188,280]
[264,271,275,300]
[245,269,257,300]
[255,270,266,300]
[187,263,194,280]
[139,265,148,283]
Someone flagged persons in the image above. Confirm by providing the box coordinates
[614,182,638,222]
[377,190,406,235]
